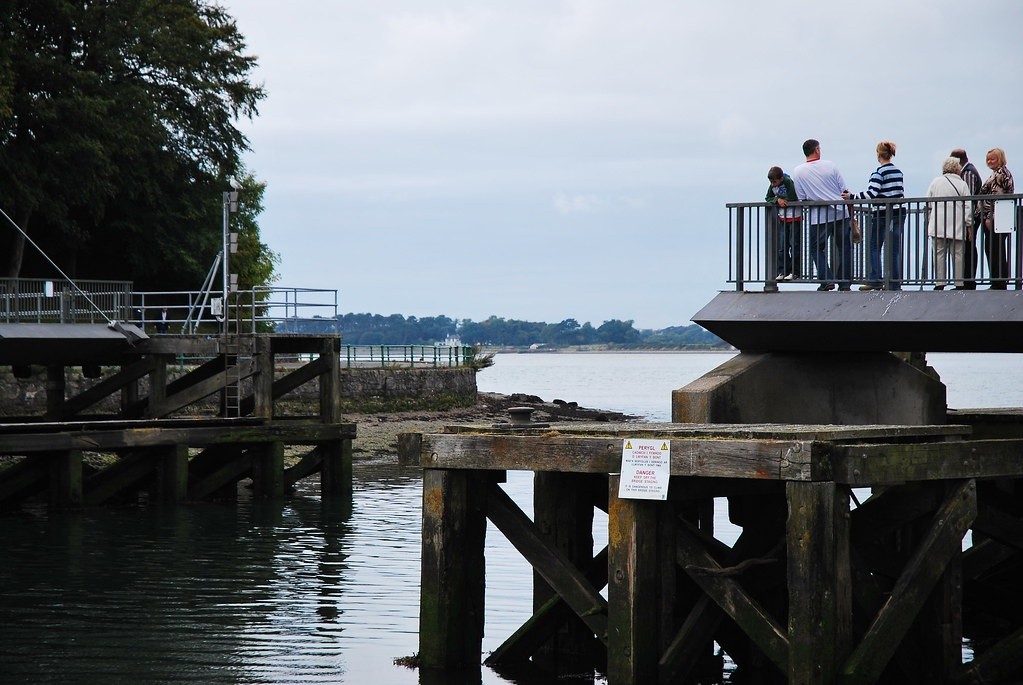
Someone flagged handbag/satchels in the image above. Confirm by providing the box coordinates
[852,218,861,243]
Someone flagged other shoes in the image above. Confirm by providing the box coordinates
[951,281,976,290]
[934,285,944,290]
[818,282,835,291]
[838,286,850,291]
[859,283,882,291]
[990,283,1007,290]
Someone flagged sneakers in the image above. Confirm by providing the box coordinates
[776,272,784,279]
[784,272,800,280]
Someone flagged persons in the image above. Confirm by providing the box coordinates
[841,140,904,291]
[948,147,983,289]
[793,138,855,291]
[978,147,1015,290]
[927,156,974,290]
[765,165,804,280]
